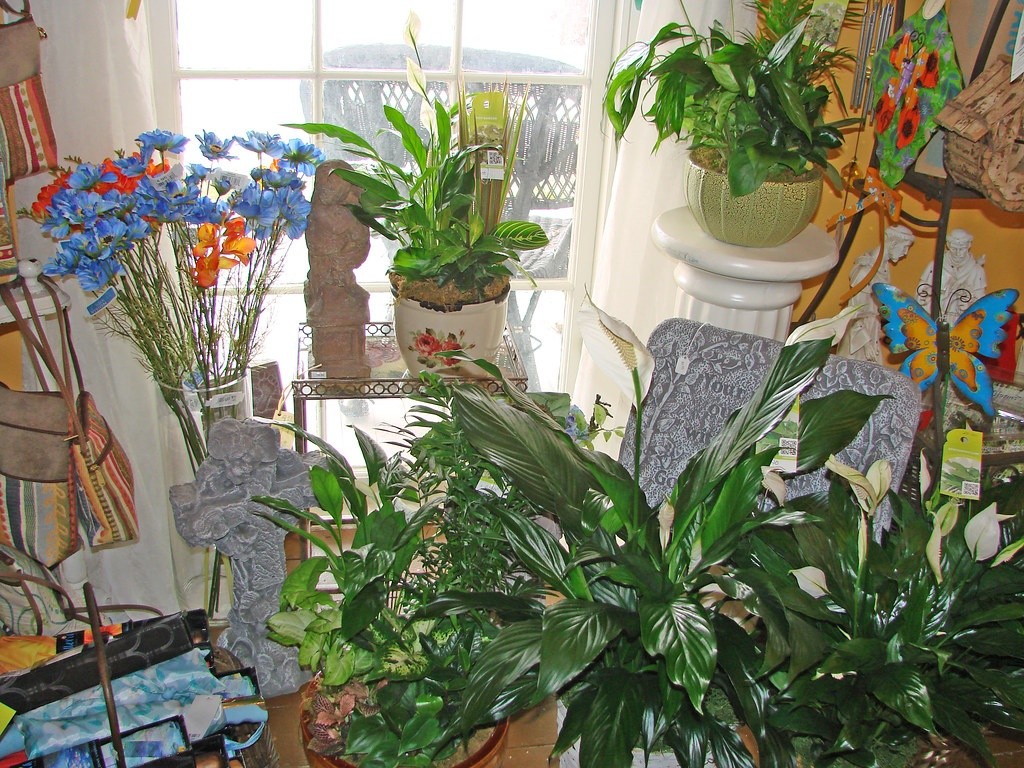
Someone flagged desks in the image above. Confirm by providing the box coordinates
[292,317,527,565]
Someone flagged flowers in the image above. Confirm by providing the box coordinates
[435,290,1024,768]
[14,124,327,621]
[280,9,550,295]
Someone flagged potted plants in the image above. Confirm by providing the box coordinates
[249,364,539,768]
[602,0,872,249]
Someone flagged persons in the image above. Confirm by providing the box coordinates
[918,228,986,323]
[837,224,914,364]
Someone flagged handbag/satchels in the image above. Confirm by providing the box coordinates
[0,270,140,643]
[0,0,58,270]
[935,55,1024,213]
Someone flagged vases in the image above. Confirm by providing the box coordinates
[390,287,512,377]
[550,698,684,768]
[162,368,253,627]
